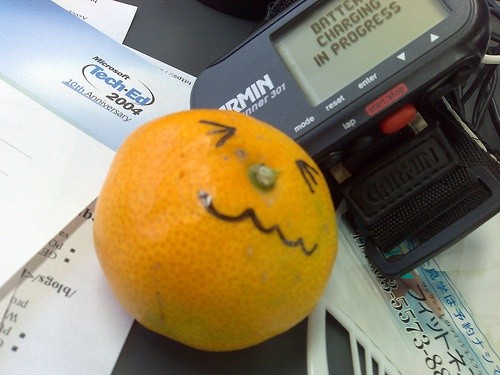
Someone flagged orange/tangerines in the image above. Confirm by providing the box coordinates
[93,108,339,352]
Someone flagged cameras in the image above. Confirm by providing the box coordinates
[189,0,500,278]
